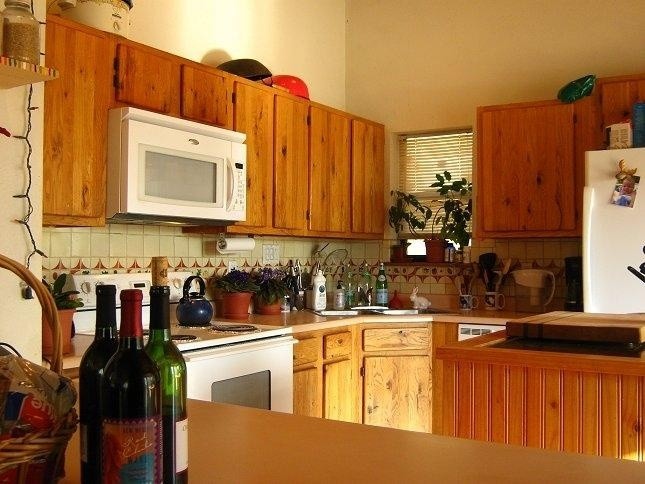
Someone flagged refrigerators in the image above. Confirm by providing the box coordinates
[582,148,645,315]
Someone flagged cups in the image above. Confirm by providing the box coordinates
[484,291,505,311]
[459,295,479,310]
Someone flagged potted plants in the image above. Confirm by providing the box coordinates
[387,169,474,262]
[36,272,84,356]
[255,267,288,313]
[215,270,258,319]
[391,238,414,264]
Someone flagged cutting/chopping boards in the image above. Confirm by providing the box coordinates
[505,312,645,349]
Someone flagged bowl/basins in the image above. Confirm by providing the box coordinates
[265,74,311,98]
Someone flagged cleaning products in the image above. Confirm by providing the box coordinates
[311,269,327,311]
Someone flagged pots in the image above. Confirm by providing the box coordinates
[214,58,273,88]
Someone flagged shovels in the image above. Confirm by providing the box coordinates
[480,252,497,293]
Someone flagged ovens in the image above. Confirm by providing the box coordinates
[109,106,247,223]
[184,337,300,414]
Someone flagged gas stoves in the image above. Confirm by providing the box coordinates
[71,319,291,351]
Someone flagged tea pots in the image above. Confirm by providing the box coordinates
[176,275,214,325]
[509,268,555,314]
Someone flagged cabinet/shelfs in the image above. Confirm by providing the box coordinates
[114,39,232,126]
[358,319,435,439]
[593,74,645,148]
[0,55,60,91]
[222,73,309,238]
[472,95,597,239]
[289,324,364,424]
[46,14,115,231]
[307,101,385,241]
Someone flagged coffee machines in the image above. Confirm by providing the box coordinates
[560,250,582,311]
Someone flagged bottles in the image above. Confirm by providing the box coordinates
[144,255,189,483]
[101,288,165,484]
[78,285,118,484]
[376,262,388,306]
[360,263,372,306]
[343,264,357,308]
